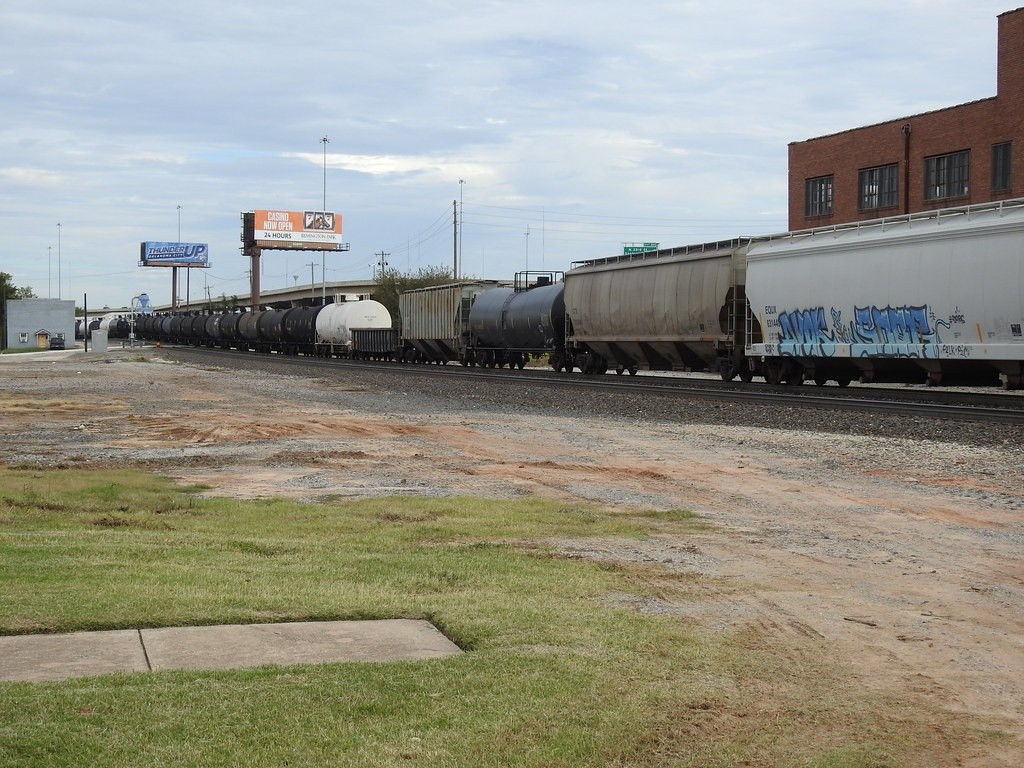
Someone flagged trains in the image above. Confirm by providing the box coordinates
[74,196,1024,393]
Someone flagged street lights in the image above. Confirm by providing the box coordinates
[319,133,331,305]
[57,221,62,298]
[47,245,52,295]
[176,203,184,305]
[455,177,467,279]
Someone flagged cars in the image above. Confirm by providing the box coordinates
[48,338,64,350]
